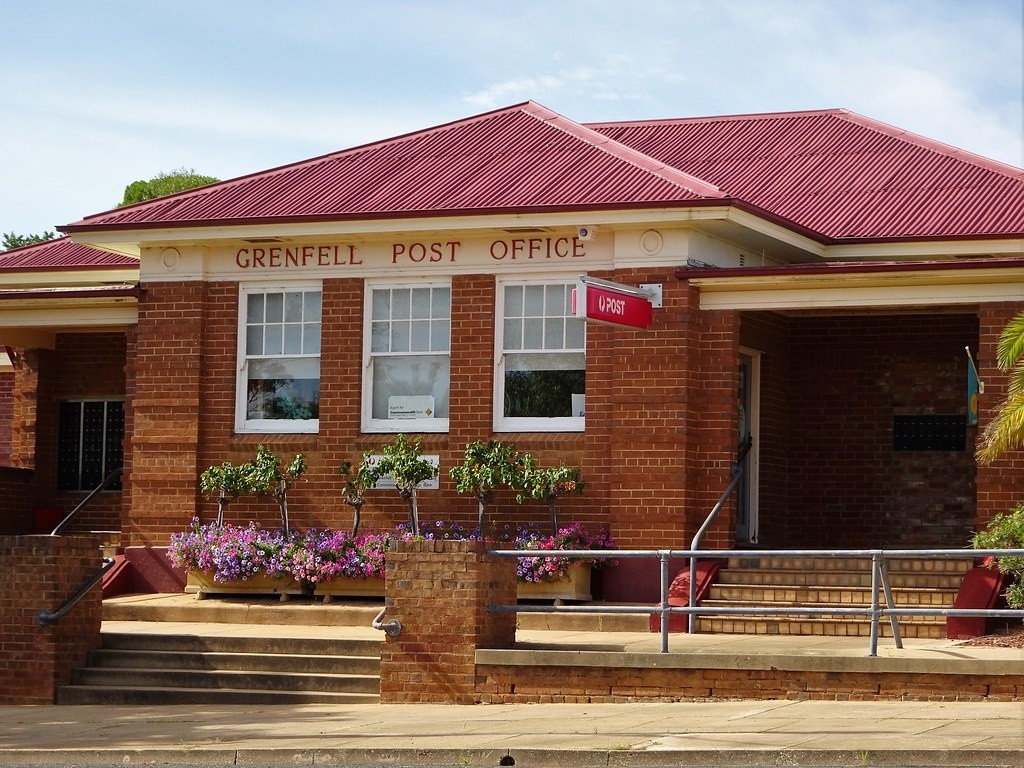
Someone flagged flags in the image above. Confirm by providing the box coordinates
[968,359,977,424]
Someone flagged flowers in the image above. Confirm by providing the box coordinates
[164,448,313,576]
[306,434,451,583]
[447,438,620,583]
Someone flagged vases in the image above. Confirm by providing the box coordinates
[517,562,592,605]
[180,569,306,602]
[313,577,385,604]
